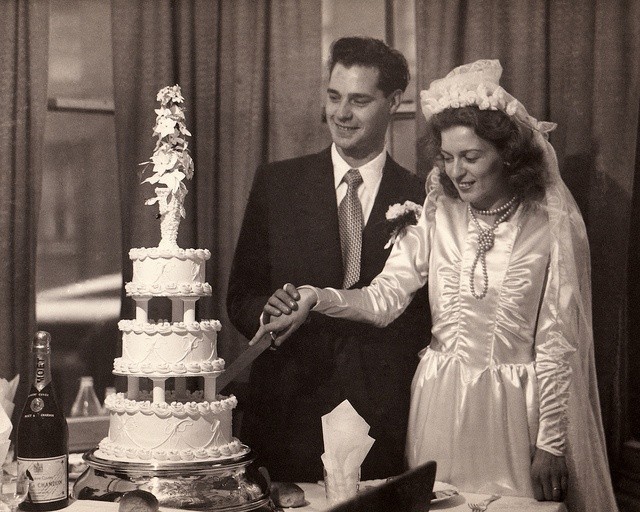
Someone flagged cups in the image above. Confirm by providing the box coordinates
[324,466,361,508]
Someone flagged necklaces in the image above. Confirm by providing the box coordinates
[468,194,520,300]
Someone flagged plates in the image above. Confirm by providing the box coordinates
[356,476,455,503]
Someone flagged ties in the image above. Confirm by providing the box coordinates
[337,170,364,290]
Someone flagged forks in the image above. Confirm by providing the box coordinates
[467,494,501,511]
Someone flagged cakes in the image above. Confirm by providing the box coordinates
[92,237,251,465]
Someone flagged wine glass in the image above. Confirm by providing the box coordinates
[0,463,30,512]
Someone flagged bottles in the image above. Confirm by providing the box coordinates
[16,329,69,511]
[69,374,103,417]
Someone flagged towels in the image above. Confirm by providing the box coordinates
[0,375,20,466]
[318,397,376,504]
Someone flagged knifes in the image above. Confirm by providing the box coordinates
[217,331,282,394]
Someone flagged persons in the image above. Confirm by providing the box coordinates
[250,60,618,511]
[226,38,432,481]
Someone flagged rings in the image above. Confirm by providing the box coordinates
[550,484,563,494]
